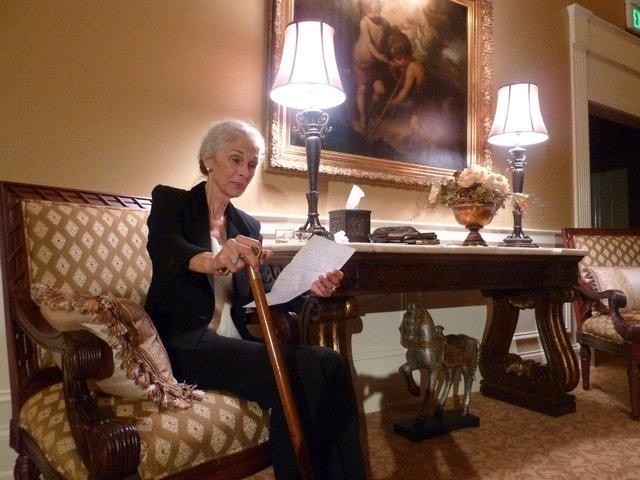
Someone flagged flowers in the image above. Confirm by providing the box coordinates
[427,166,544,218]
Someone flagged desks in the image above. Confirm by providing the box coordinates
[259,241,590,477]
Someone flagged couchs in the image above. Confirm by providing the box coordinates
[562,227,639,423]
[0,179,364,477]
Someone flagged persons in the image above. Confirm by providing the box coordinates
[145,117,366,480]
[344,1,431,122]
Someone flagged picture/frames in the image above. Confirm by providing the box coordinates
[263,0,494,190]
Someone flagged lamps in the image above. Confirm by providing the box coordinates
[486,83,550,244]
[268,21,347,240]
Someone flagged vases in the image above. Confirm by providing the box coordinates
[447,204,497,246]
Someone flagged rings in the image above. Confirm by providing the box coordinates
[327,283,336,292]
[231,257,240,264]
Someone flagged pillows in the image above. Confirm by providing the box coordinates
[29,279,205,409]
[583,266,640,312]
